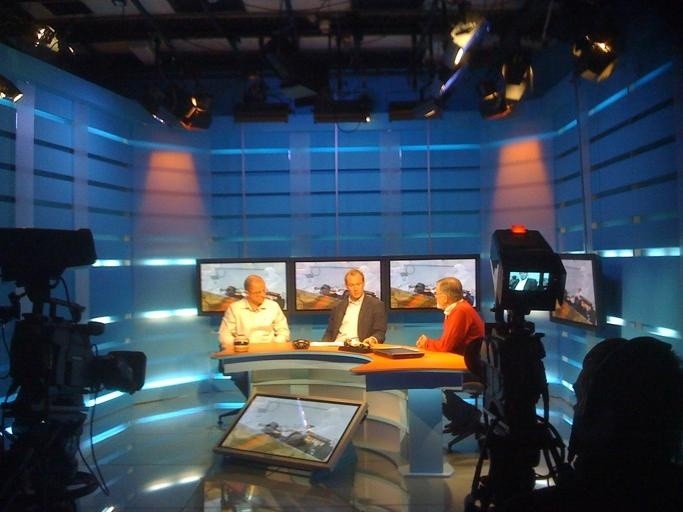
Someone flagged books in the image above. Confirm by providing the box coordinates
[372,345,424,359]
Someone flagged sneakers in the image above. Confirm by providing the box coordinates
[444,406,482,436]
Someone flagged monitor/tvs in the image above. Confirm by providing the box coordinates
[550,253,602,329]
[196,257,291,316]
[291,256,385,315]
[385,254,480,312]
[213,391,369,473]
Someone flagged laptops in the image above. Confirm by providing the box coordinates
[374,348,424,358]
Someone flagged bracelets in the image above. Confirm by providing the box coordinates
[371,338,376,344]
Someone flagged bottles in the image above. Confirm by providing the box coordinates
[233,333,250,354]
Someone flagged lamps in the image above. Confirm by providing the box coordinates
[231,22,612,119]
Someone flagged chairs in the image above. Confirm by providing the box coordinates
[441,373,488,458]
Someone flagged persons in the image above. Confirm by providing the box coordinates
[511,271,535,292]
[216,275,291,400]
[320,270,387,346]
[414,276,485,437]
[494,337,682,512]
